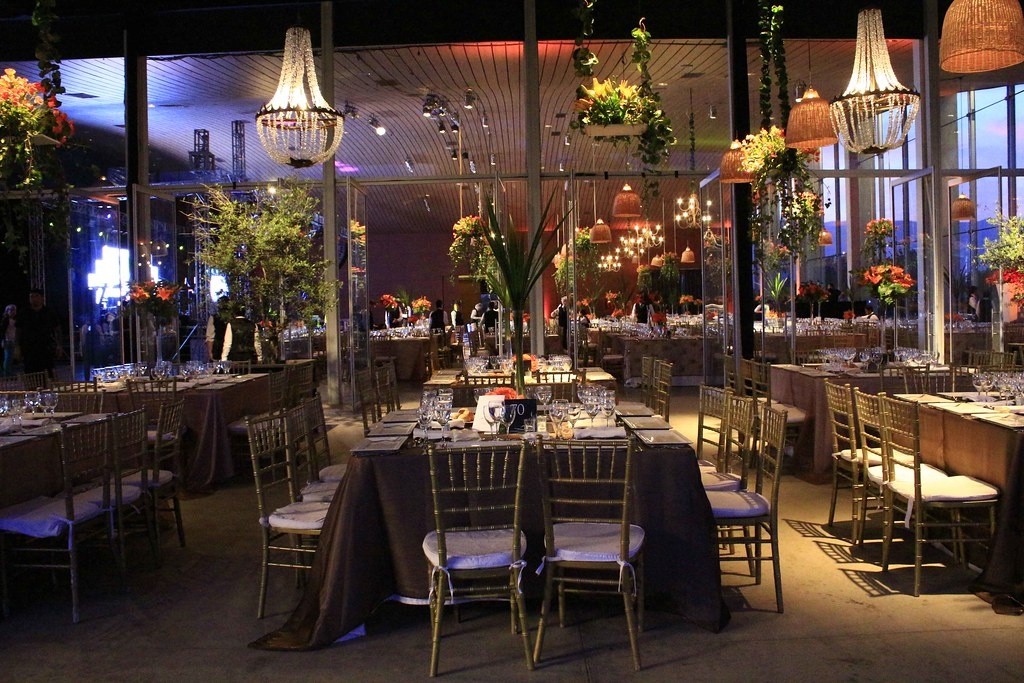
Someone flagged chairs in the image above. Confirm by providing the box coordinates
[0,329,1024,675]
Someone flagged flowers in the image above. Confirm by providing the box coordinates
[129,81,1023,316]
[0,62,76,185]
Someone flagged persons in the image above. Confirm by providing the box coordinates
[864,305,879,320]
[79,312,121,381]
[578,310,590,327]
[0,304,18,377]
[470,303,484,322]
[450,304,464,328]
[206,296,263,365]
[121,296,129,310]
[629,290,668,323]
[480,302,498,332]
[551,296,568,352]
[967,286,995,322]
[353,300,380,332]
[754,300,771,320]
[384,303,411,329]
[826,283,835,292]
[428,300,449,331]
[15,288,64,382]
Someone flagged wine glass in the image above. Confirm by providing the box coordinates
[417,384,615,447]
[819,346,939,376]
[599,317,685,339]
[285,327,324,339]
[371,328,428,339]
[466,355,572,375]
[0,391,58,432]
[972,371,1024,407]
[91,360,231,385]
[767,318,991,333]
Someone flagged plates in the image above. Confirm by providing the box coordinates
[197,373,269,389]
[0,414,123,446]
[350,410,421,451]
[895,393,1024,428]
[772,365,835,377]
[615,404,693,444]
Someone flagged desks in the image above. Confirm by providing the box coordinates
[0,319,1024,649]
[36,368,185,472]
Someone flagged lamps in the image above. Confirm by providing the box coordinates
[255,22,344,167]
[346,1,779,299]
[951,78,978,219]
[937,0,1024,74]
[831,9,924,157]
[816,225,832,246]
[785,41,839,149]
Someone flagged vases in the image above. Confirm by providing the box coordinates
[993,281,1024,324]
[573,116,646,144]
[154,323,181,369]
